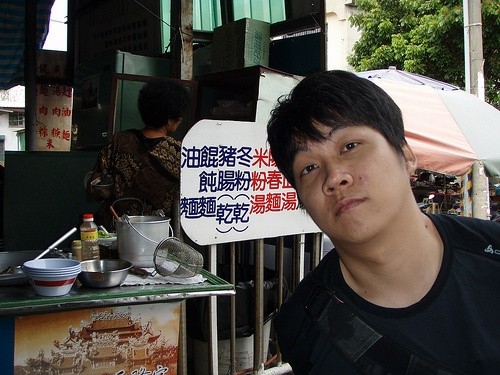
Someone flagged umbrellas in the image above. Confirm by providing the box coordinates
[354,66,500,178]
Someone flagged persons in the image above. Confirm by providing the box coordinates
[86,83,190,238]
[268,70,500,375]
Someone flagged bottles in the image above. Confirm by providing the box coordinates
[72,239,82,260]
[80,213,101,260]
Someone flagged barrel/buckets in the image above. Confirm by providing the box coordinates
[115,215,173,268]
[0,247,67,285]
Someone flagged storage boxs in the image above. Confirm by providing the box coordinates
[214,18,271,68]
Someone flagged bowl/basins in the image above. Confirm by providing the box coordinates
[23,258,82,297]
[77,259,134,287]
[98,233,124,249]
